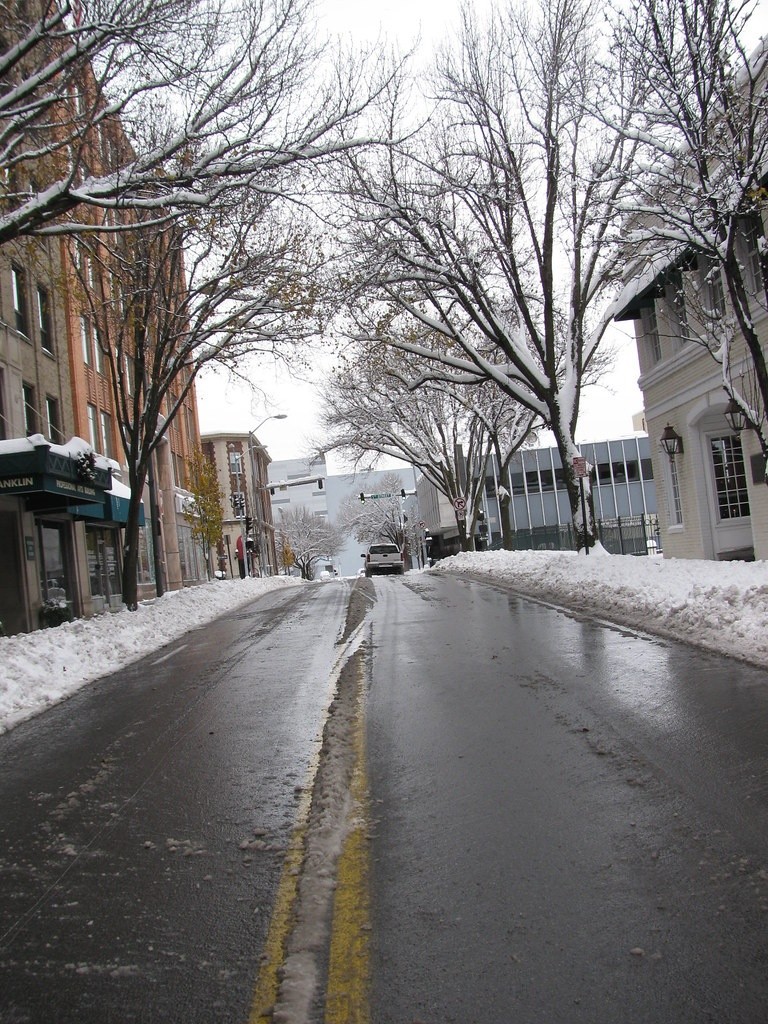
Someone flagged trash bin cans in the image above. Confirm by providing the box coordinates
[40,600,76,626]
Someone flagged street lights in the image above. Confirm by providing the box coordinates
[248,413,286,578]
[234,444,267,579]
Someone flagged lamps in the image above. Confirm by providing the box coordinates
[659,421,684,464]
[723,397,755,440]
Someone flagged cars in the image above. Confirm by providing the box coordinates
[357,568,367,577]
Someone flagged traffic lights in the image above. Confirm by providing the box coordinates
[239,498,245,509]
[233,495,240,508]
[401,489,406,501]
[360,492,365,504]
[245,516,253,534]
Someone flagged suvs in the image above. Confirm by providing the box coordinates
[359,542,405,578]
